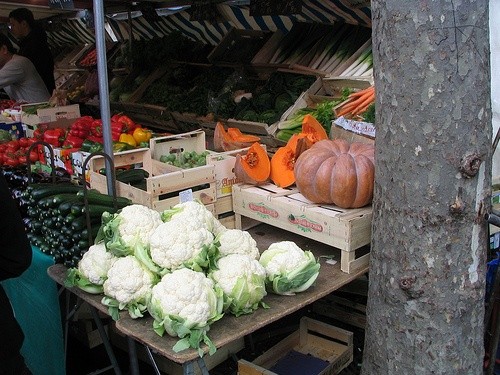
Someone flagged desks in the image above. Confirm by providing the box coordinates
[48,224,370,375]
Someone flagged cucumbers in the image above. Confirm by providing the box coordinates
[19,167,175,269]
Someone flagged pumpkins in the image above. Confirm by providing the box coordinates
[213,116,375,210]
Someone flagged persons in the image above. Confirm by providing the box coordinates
[0,30,50,104]
[0,173,33,375]
[9,9,56,97]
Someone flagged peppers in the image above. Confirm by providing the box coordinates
[43,115,176,169]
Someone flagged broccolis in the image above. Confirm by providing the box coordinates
[65,199,321,353]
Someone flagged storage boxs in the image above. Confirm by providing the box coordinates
[145,336,245,375]
[90,130,267,231]
[232,181,372,274]
[41,20,376,159]
[21,102,91,189]
[238,317,353,375]
[0,121,23,140]
[313,275,369,330]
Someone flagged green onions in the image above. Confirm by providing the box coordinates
[312,27,373,78]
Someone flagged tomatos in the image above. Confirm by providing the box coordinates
[0,130,43,165]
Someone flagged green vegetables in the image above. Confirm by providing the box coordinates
[276,89,374,141]
